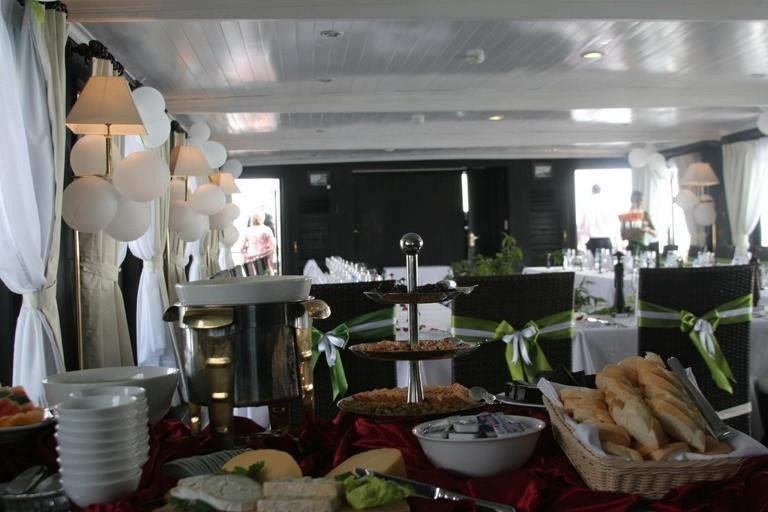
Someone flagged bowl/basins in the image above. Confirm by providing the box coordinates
[42,366,180,507]
[412,415,546,474]
[174,273,314,304]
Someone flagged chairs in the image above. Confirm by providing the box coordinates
[451,274,575,391]
[637,267,753,438]
[310,280,396,415]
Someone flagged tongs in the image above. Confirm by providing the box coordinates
[667,354,731,441]
[353,465,518,512]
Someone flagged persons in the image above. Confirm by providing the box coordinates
[579,185,618,252]
[621,190,658,257]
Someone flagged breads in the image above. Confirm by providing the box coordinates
[256,499,343,511]
[170,473,262,512]
[261,476,345,498]
[555,356,735,461]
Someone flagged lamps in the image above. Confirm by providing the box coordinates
[65,74,147,181]
[461,173,470,213]
[169,144,212,202]
[681,163,720,203]
[464,48,485,64]
[210,173,241,200]
[411,114,425,123]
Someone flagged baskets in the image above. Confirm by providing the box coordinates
[542,391,751,500]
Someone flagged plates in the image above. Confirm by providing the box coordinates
[0,415,56,446]
[165,445,255,481]
[496,392,545,408]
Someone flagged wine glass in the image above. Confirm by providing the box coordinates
[632,268,640,292]
[325,255,371,283]
[561,247,717,274]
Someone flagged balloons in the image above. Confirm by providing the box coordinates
[628,144,665,168]
[678,190,716,225]
[62,86,244,248]
[757,111,768,135]
